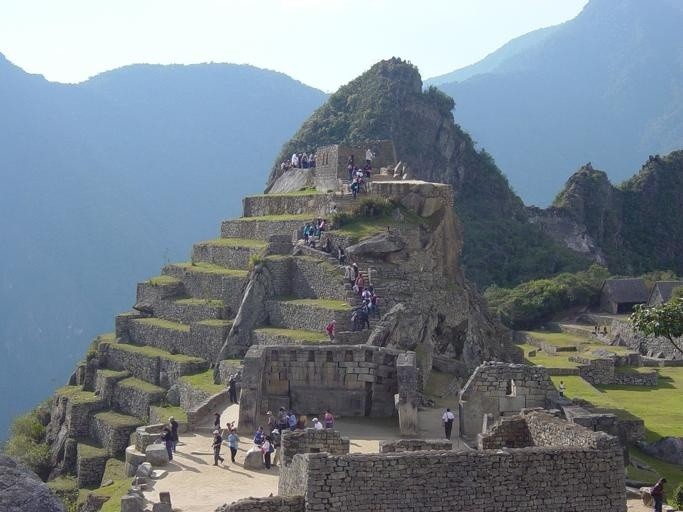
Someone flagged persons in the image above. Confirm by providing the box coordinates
[650,478,666,512]
[347,155,371,200]
[225,374,239,404]
[212,412,220,428]
[228,428,239,463]
[253,405,333,471]
[279,152,316,171]
[325,319,336,343]
[224,422,232,440]
[558,379,564,396]
[160,426,173,462]
[365,148,375,165]
[441,407,454,442]
[303,216,377,331]
[212,429,224,467]
[168,415,178,451]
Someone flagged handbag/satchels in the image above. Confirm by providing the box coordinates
[447,418,453,423]
[269,445,274,452]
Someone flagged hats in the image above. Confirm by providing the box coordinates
[213,430,219,434]
[312,418,318,422]
[266,411,273,415]
[271,429,280,435]
